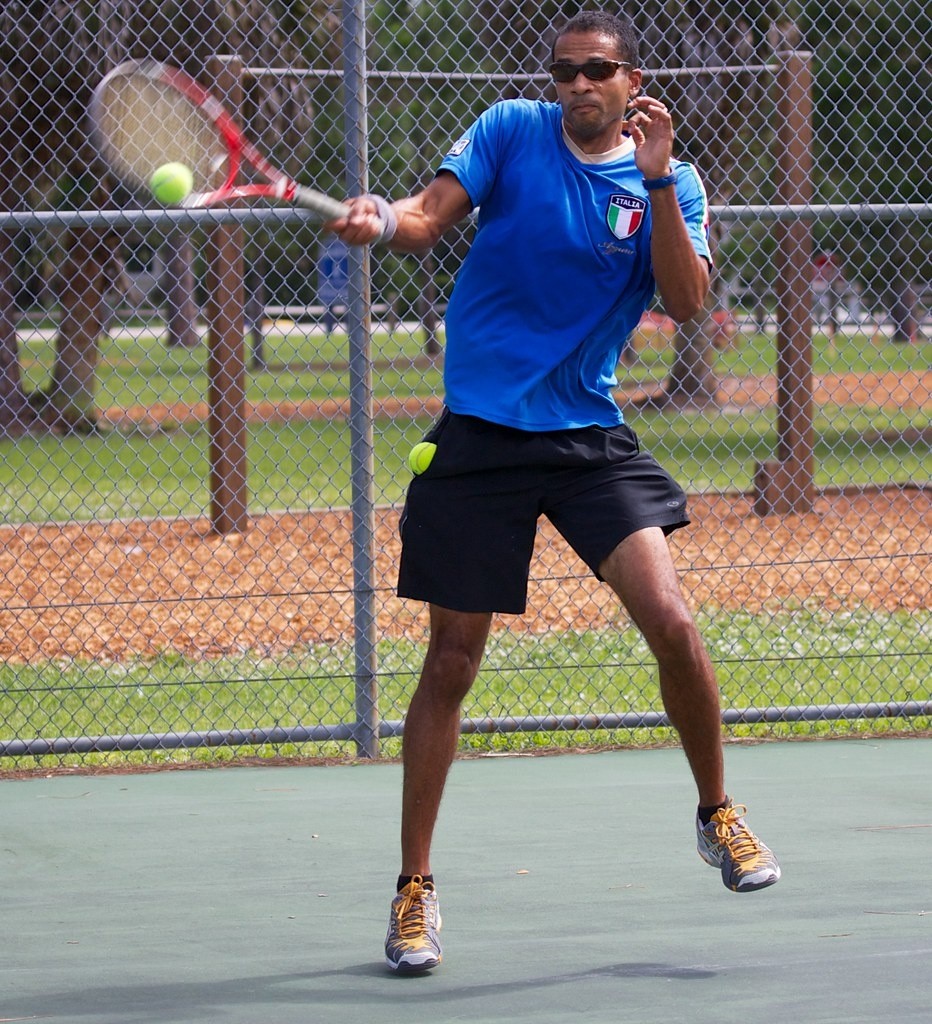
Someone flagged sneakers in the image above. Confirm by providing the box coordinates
[382,874,444,975]
[695,796,781,893]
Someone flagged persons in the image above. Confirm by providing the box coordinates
[325,12,784,973]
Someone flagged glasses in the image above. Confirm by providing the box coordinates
[547,59,633,84]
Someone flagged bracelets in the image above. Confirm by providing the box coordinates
[364,192,397,244]
[641,167,678,191]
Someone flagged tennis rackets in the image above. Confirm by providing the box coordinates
[84,56,352,219]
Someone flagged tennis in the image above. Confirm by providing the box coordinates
[148,160,195,206]
[407,439,439,476]
